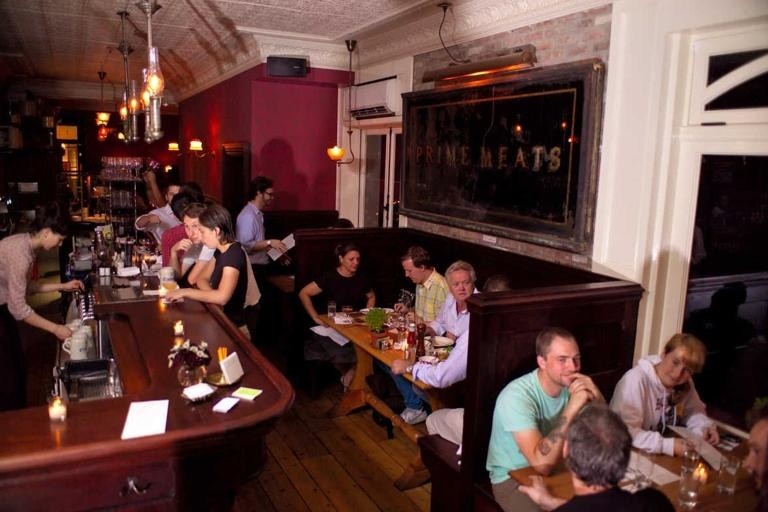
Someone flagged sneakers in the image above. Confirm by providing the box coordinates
[392,408,427,427]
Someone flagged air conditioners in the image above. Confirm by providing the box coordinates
[344,79,397,120]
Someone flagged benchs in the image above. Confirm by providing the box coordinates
[462,471,504,512]
[417,434,462,512]
[277,304,386,401]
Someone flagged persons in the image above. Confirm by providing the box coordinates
[393,245,450,424]
[133,169,289,341]
[609,333,720,457]
[415,261,482,338]
[391,273,516,445]
[485,323,607,511]
[299,242,376,392]
[744,403,768,496]
[518,403,676,512]
[0,206,87,410]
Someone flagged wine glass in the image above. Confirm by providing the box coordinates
[162,277,177,304]
[343,306,353,323]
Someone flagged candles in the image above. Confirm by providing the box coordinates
[49,398,66,421]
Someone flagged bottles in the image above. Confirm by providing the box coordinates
[81,207,88,223]
[140,158,143,169]
[126,192,134,212]
[404,323,418,360]
[397,323,406,342]
[139,160,147,178]
[101,156,139,179]
[95,230,110,265]
[119,191,125,214]
[112,191,118,214]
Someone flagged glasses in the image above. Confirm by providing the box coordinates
[265,191,273,195]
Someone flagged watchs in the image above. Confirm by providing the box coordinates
[267,238,271,246]
[404,363,415,374]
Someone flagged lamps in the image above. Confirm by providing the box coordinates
[190,140,215,159]
[94,0,165,142]
[421,52,536,83]
[168,142,190,159]
[327,40,358,167]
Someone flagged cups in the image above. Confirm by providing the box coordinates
[681,438,701,471]
[65,333,89,351]
[157,267,176,286]
[69,320,82,332]
[327,302,337,319]
[62,339,87,360]
[79,326,94,348]
[717,453,740,494]
[678,469,700,508]
[636,451,654,487]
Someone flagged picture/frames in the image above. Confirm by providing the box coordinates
[398,58,606,255]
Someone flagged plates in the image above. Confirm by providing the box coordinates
[360,308,394,314]
[419,356,437,365]
[424,336,454,346]
